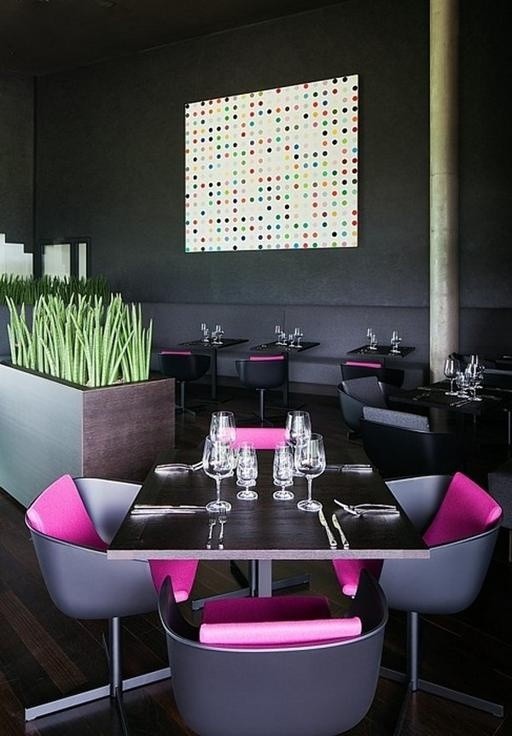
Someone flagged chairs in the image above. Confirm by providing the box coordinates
[25,343,512,736]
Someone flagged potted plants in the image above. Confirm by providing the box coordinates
[0,289,179,506]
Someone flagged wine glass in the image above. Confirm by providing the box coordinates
[367,328,402,353]
[275,325,302,348]
[199,410,327,515]
[200,322,225,345]
[443,351,484,402]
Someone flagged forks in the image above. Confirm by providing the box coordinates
[206,509,216,549]
[217,507,227,549]
[333,499,399,518]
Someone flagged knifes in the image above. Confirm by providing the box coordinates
[331,513,350,547]
[325,464,373,472]
[317,509,338,546]
[130,504,207,516]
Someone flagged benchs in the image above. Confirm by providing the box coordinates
[1,301,512,395]
